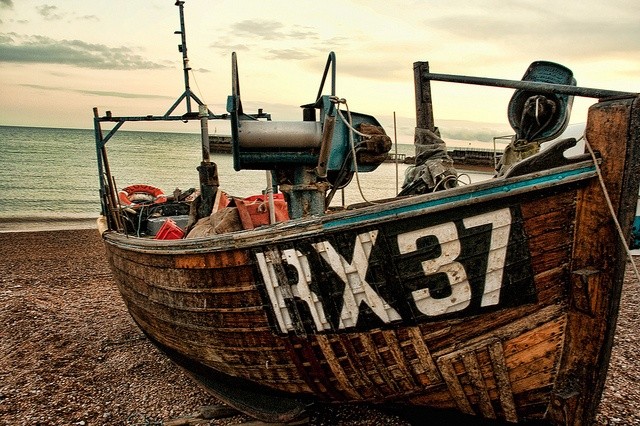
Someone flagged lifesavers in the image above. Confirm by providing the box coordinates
[118,184,168,209]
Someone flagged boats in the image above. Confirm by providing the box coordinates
[93,0,640,424]
